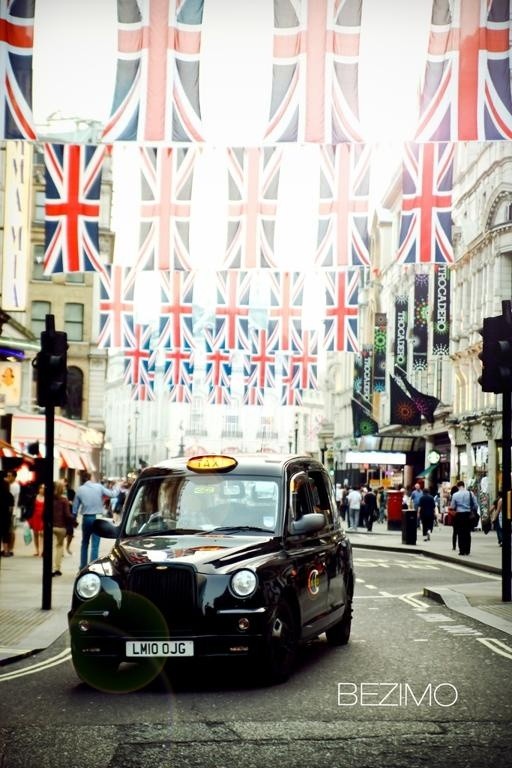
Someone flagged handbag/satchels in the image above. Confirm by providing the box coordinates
[469,491,479,528]
[62,502,73,536]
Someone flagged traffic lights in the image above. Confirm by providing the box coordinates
[41,335,66,402]
[492,329,510,394]
[476,317,497,393]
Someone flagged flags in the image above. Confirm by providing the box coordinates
[1,1,35,142]
[414,1,511,143]
[268,2,365,148]
[99,2,209,143]
[1,1,512,413]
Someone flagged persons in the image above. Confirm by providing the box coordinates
[1,432,512,577]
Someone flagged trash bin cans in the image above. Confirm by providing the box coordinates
[387,490,404,531]
[401,510,418,545]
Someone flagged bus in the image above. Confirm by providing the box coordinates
[332,452,406,520]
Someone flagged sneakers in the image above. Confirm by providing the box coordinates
[51,571,61,577]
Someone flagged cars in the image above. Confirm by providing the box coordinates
[67,452,354,688]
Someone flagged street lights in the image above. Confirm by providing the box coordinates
[126,420,133,472]
[293,420,299,453]
[164,434,170,460]
[179,423,185,455]
[320,438,326,465]
[287,436,293,453]
[135,407,140,475]
